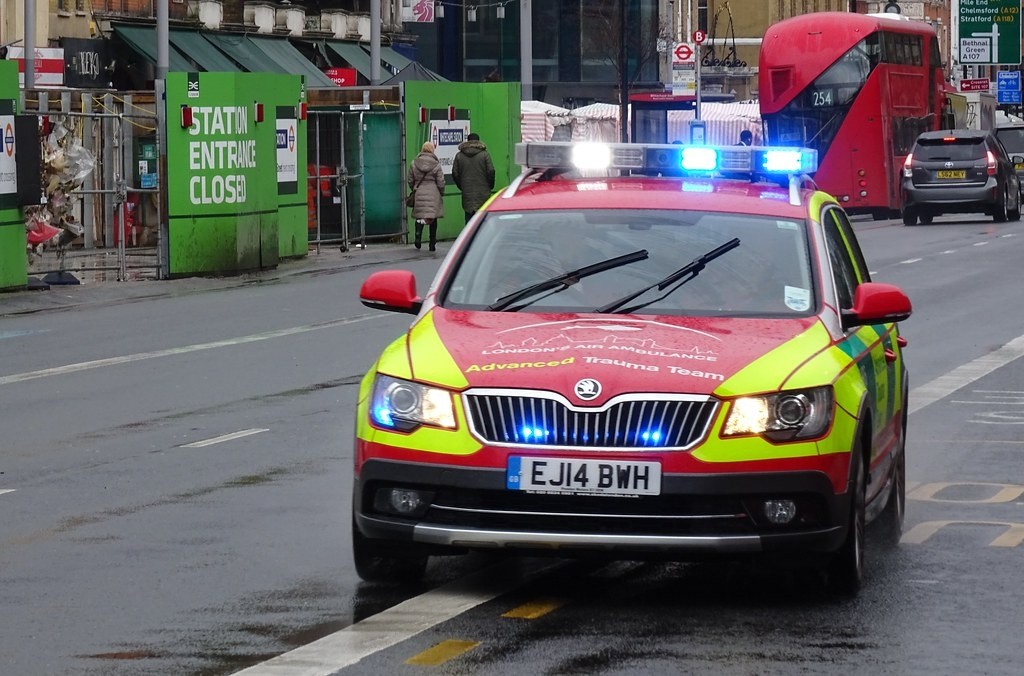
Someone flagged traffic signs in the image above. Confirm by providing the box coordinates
[958,0,1022,66]
[960,78,990,92]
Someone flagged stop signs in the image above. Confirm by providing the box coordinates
[672,43,695,65]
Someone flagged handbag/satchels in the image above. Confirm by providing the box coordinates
[406,189,417,207]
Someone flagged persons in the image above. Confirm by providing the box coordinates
[722,130,754,184]
[452,133,496,226]
[308,159,336,241]
[662,139,686,177]
[408,141,447,251]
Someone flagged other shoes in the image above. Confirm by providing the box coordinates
[429,245,436,251]
[414,232,422,249]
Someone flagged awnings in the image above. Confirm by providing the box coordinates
[112,24,452,88]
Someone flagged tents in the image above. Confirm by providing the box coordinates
[520,100,771,182]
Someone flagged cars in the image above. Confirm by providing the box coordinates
[352,141,912,591]
[900,127,1024,226]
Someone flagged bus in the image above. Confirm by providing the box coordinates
[758,12,953,222]
